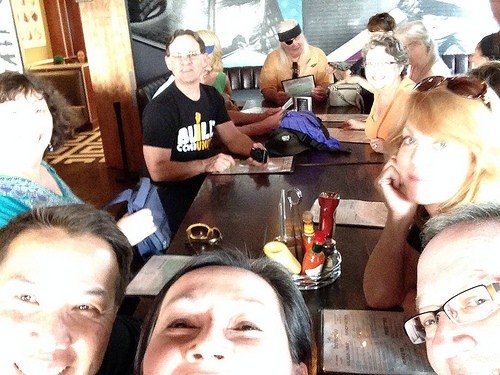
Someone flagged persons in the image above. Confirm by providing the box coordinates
[131,240,319,375]
[361,68,499,374]
[331,9,500,153]
[53,49,86,65]
[1,200,133,375]
[1,67,158,248]
[256,15,335,106]
[140,29,286,183]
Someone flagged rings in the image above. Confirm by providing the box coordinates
[374,143,378,148]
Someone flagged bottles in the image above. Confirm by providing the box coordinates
[300,191,341,276]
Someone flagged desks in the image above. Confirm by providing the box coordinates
[220,99,385,166]
[130,163,421,349]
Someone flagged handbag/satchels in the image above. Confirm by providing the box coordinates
[281,111,354,154]
[128,177,171,255]
[328,81,362,107]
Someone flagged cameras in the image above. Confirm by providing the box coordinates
[250,147,267,164]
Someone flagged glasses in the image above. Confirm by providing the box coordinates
[363,60,402,70]
[187,223,222,255]
[403,282,500,345]
[167,52,203,61]
[414,75,492,110]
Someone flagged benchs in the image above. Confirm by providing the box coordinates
[136,54,474,133]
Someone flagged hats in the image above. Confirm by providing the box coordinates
[267,132,310,155]
